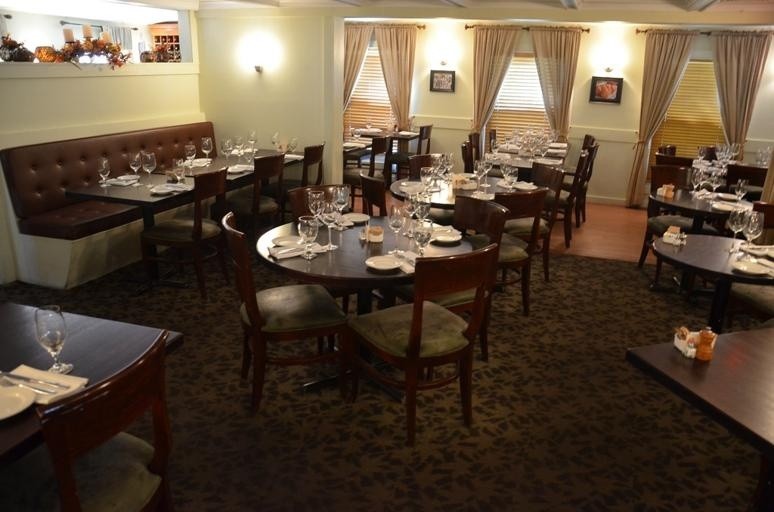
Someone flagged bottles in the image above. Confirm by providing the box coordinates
[359,225,385,244]
[683,325,716,362]
[661,183,677,199]
[673,231,686,248]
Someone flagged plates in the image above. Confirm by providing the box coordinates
[272,235,302,247]
[342,211,371,224]
[434,234,462,243]
[364,255,403,270]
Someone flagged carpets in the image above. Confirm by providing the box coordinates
[0,247,774,512]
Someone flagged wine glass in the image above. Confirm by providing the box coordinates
[297,186,350,260]
[689,140,773,278]
[388,192,432,267]
[418,126,562,202]
[34,305,73,375]
[89,127,297,194]
[341,119,413,148]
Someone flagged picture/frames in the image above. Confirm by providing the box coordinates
[588,75,623,104]
[429,68,455,92]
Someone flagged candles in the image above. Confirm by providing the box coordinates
[63,24,112,44]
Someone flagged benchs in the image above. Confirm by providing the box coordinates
[0,112,279,290]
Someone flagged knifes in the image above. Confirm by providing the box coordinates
[0,372,70,389]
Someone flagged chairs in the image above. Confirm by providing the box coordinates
[0,327,175,512]
[637,142,774,286]
